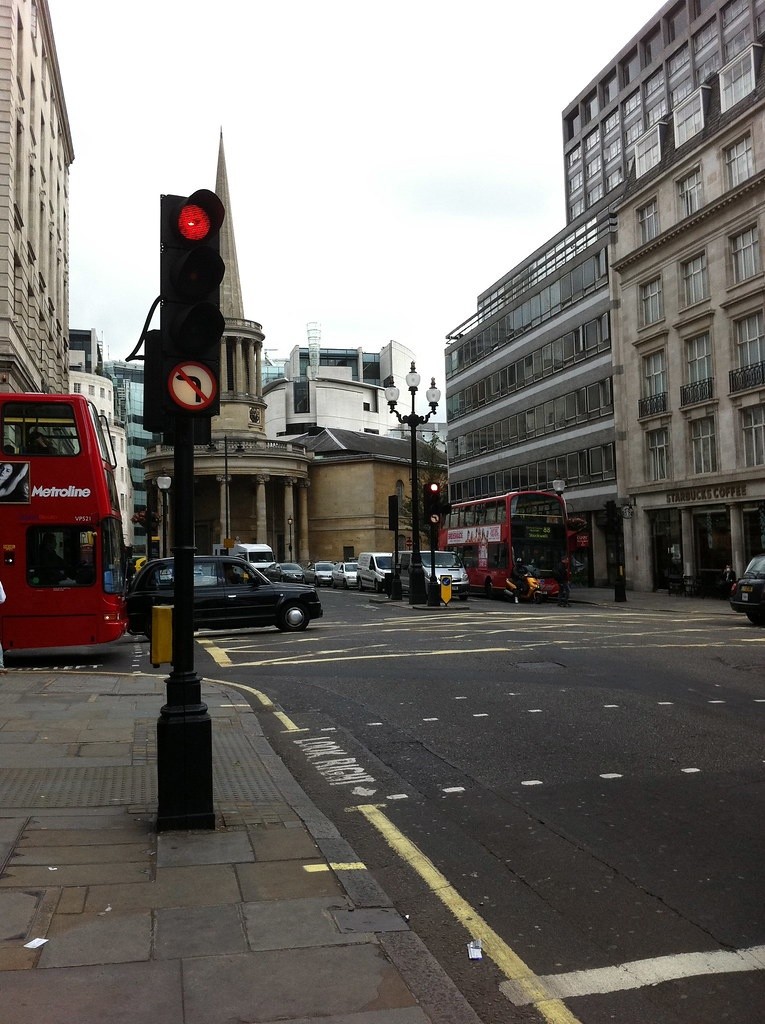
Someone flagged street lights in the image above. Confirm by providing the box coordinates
[288,515,294,562]
[157,467,172,558]
[384,362,440,603]
[553,475,565,516]
[206,435,246,556]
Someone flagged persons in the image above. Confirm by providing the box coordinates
[506,558,529,604]
[527,557,542,579]
[0,581,7,675]
[224,563,245,586]
[722,565,737,601]
[29,533,67,586]
[556,557,571,608]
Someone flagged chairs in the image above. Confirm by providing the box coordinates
[4,444,15,454]
[668,573,721,600]
[19,445,28,455]
[29,529,62,585]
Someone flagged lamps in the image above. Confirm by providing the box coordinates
[2,375,7,383]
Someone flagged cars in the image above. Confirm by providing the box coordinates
[128,555,324,639]
[304,563,335,587]
[262,562,305,584]
[729,552,765,627]
[331,562,358,590]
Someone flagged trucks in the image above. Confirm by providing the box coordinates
[229,543,276,578]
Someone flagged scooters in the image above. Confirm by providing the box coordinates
[503,571,543,604]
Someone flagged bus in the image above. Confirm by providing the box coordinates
[437,491,576,600]
[0,391,128,650]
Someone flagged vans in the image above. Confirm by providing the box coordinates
[391,550,470,600]
[357,552,393,593]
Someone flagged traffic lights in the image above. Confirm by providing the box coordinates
[440,503,452,515]
[161,188,227,365]
[603,501,615,529]
[425,482,441,514]
